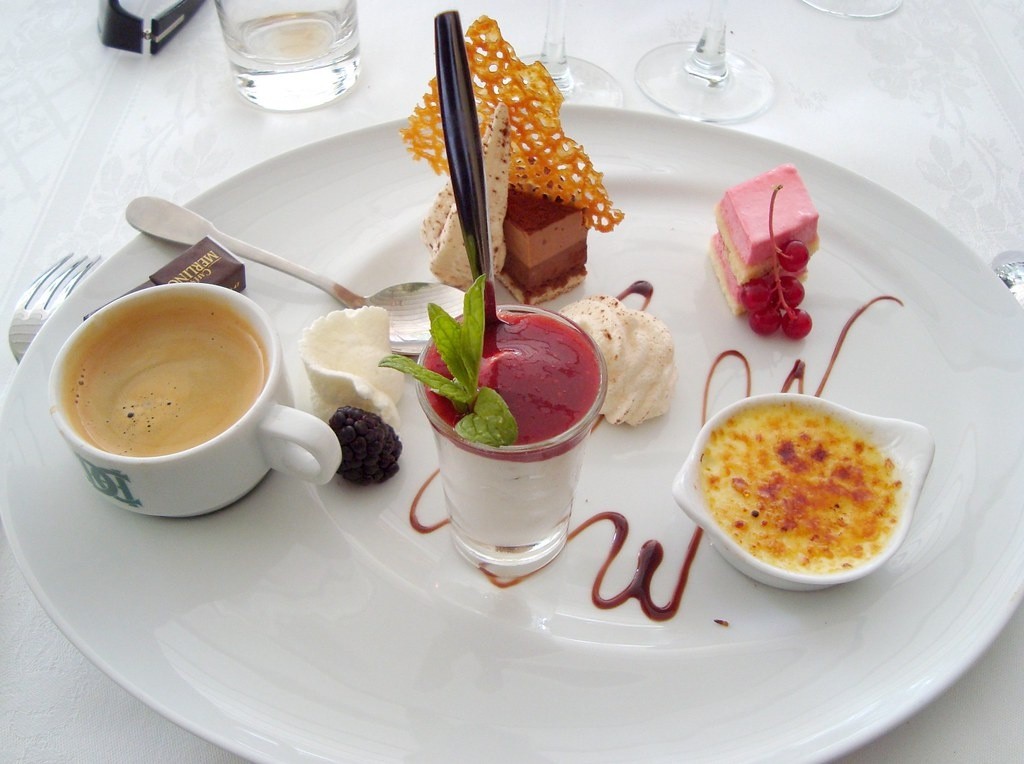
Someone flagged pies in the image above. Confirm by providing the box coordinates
[419,103,512,288]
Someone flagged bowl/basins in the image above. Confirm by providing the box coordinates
[673,396,932,594]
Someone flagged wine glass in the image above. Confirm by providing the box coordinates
[804,0,903,17]
[519,0,623,110]
[632,0,774,125]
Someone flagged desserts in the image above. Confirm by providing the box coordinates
[497,182,593,305]
[709,162,820,314]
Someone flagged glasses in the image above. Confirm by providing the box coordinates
[96,0,205,54]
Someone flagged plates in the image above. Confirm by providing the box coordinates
[0,110,1024,764]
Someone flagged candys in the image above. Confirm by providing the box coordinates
[80,236,248,320]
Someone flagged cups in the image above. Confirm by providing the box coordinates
[50,284,345,520]
[419,305,610,580]
[215,0,363,110]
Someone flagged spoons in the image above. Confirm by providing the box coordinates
[121,196,466,342]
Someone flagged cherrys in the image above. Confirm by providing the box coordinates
[737,184,812,340]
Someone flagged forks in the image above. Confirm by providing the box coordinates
[8,253,102,362]
